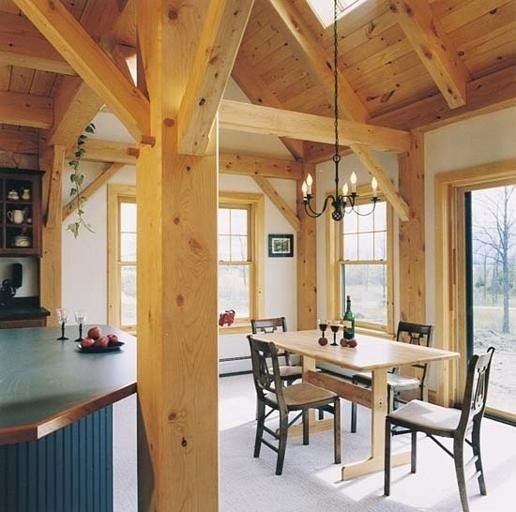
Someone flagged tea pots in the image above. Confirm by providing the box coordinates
[6,209,26,224]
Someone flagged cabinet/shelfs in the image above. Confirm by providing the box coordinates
[0,167,46,257]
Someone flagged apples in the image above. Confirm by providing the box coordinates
[81,327,118,348]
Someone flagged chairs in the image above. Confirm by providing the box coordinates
[384,346,495,512]
[246,334,341,475]
[251,317,323,420]
[351,320,433,433]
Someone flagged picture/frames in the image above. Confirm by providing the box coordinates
[268,233,294,256]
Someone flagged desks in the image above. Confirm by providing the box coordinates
[251,329,460,482]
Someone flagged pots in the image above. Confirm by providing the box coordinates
[13,235,32,247]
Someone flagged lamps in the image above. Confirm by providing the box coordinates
[301,0,381,222]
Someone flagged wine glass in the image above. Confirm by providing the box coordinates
[329,320,342,347]
[72,307,88,342]
[53,308,70,342]
[317,320,328,339]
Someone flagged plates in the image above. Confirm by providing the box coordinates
[78,342,125,352]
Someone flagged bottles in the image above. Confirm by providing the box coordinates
[343,296,355,340]
[20,187,31,200]
[8,189,19,201]
